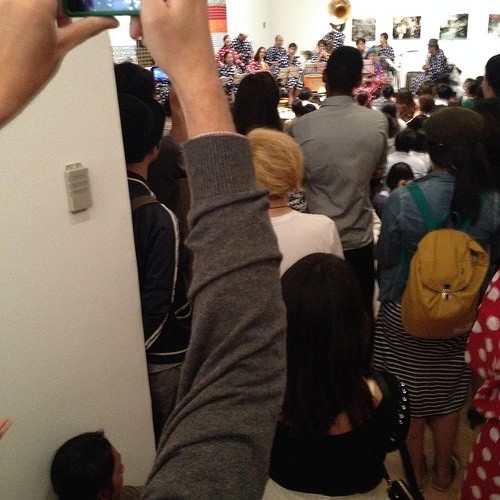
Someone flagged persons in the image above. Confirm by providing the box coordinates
[468,231,500,430]
[82,95,181,354]
[289,45,388,291]
[113,61,187,210]
[52,429,146,500]
[473,54,500,152]
[211,27,500,221]
[245,127,346,277]
[263,253,410,500]
[0,0,289,500]
[459,269,499,500]
[373,107,500,491]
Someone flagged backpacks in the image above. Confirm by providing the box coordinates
[399,182,490,340]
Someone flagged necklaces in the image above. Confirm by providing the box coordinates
[267,205,293,210]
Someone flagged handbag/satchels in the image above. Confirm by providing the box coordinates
[387,480,424,500]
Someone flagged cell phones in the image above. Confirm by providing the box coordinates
[62,0,141,16]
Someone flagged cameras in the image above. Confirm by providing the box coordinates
[152,65,170,83]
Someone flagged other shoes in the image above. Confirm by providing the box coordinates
[431,451,460,491]
